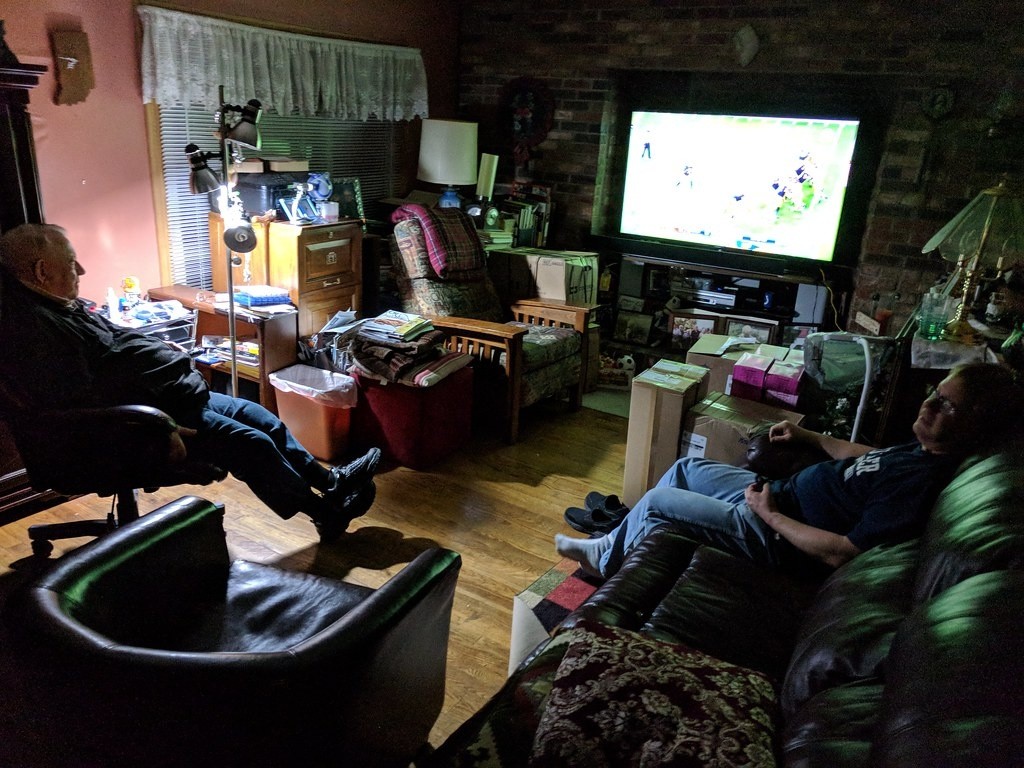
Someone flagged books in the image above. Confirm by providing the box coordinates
[192,284,298,324]
[228,157,311,173]
[491,183,551,248]
[296,309,435,373]
[476,228,514,250]
[202,334,260,368]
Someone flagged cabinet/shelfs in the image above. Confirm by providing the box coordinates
[873,301,1005,448]
[610,257,814,358]
[148,284,298,418]
[209,212,363,342]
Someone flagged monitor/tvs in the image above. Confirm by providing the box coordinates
[613,103,867,278]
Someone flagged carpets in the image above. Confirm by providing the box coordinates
[508,557,606,676]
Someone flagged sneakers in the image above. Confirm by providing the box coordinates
[310,480,376,542]
[564,507,620,535]
[584,491,629,518]
[321,447,381,497]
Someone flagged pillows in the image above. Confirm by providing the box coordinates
[530,617,778,768]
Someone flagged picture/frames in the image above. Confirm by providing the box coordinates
[722,317,775,345]
[610,308,656,347]
[666,312,718,353]
[778,322,822,348]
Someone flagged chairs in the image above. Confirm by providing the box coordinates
[1,394,228,560]
[28,495,462,768]
[388,203,600,445]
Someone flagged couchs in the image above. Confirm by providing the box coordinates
[415,434,1024,768]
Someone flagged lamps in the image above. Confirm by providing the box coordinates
[921,180,1024,346]
[417,119,478,209]
[186,85,263,399]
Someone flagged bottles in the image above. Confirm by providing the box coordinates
[985,292,1005,325]
[106,286,119,319]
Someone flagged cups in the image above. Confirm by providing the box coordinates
[921,294,953,341]
[322,202,338,223]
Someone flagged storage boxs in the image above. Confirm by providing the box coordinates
[490,246,600,394]
[622,334,806,509]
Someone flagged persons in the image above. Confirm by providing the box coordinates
[739,325,761,344]
[0,222,381,537]
[672,320,712,351]
[556,364,1011,580]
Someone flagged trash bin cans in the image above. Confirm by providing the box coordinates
[270,364,358,462]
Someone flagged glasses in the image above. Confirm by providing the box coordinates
[926,385,959,417]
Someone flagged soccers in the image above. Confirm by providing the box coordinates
[616,355,636,373]
